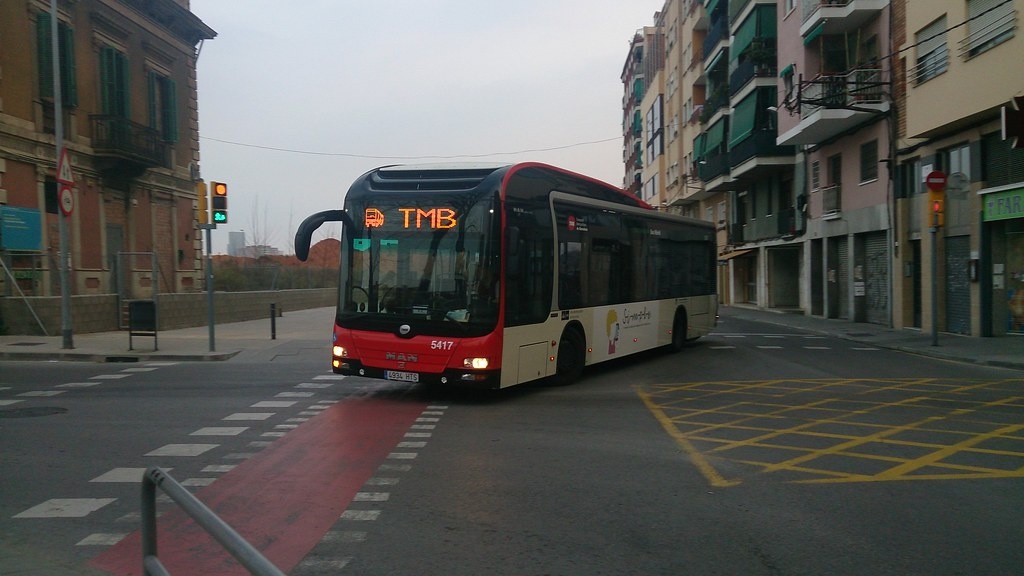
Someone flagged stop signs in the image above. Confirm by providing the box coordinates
[927,170,949,191]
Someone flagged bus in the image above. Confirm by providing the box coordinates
[294,161,717,400]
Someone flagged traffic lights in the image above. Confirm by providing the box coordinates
[210,180,229,224]
[933,200,946,212]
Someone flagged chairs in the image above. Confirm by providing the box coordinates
[435,272,468,299]
